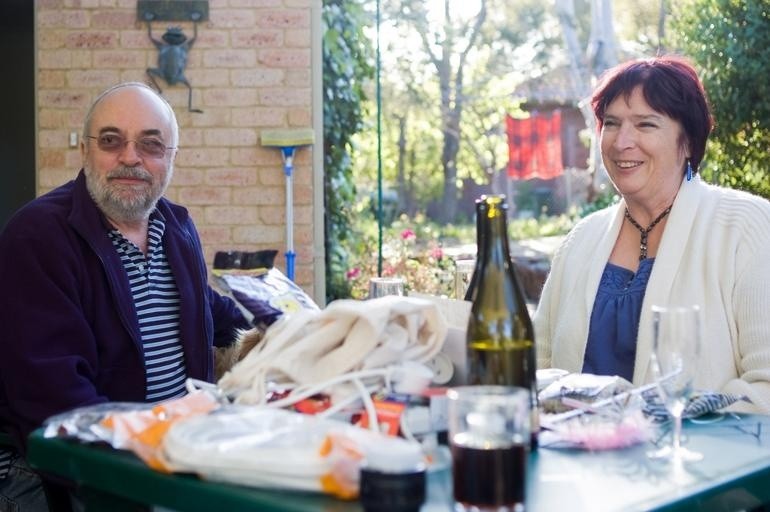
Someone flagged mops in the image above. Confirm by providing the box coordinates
[260,130,314,283]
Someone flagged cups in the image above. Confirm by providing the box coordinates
[360,464,424,511]
[368,277,405,299]
[444,381,530,509]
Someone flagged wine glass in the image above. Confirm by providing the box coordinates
[649,305,705,460]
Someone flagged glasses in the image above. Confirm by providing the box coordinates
[83,134,174,159]
[652,397,761,447]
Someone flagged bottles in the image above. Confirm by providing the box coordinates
[464,195,537,385]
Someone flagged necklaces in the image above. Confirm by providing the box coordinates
[623,206,674,264]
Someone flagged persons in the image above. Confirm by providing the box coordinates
[0,82,260,510]
[532,58,770,412]
